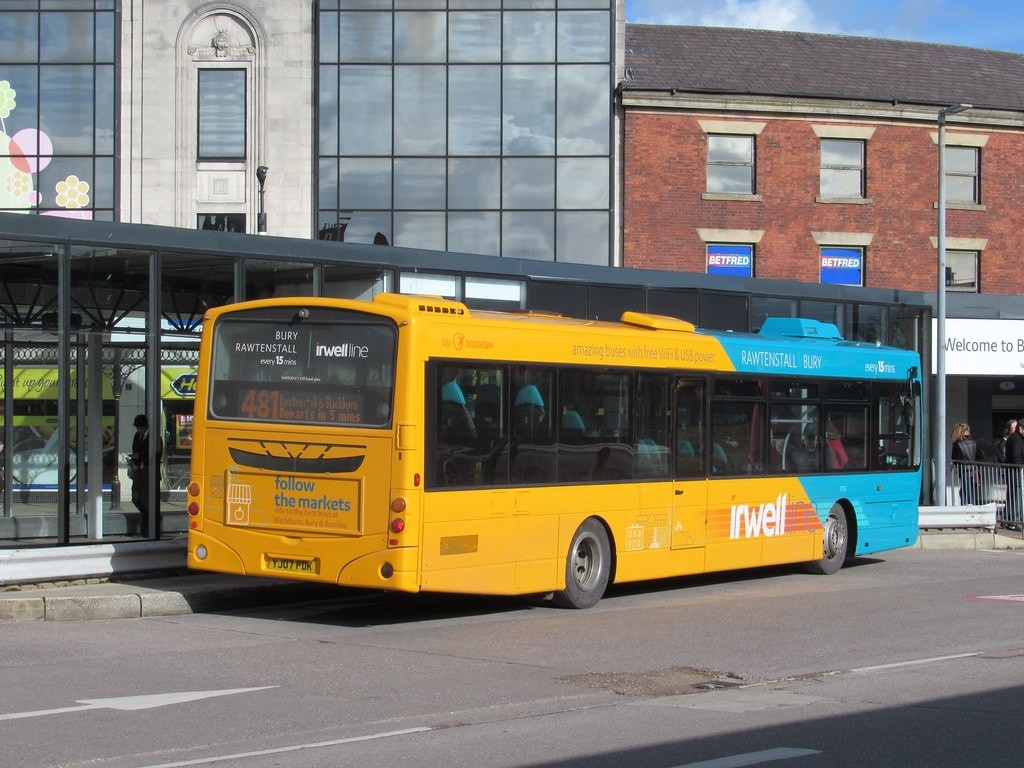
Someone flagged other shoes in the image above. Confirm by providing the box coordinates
[1007,523,1022,532]
[1000,522,1008,527]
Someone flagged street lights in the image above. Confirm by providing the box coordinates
[933,102,974,507]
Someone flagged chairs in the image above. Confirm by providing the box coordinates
[439,383,729,475]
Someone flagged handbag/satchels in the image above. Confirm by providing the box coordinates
[973,469,979,482]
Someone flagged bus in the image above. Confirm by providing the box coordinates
[187,293,926,612]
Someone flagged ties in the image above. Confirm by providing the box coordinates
[140,433,145,441]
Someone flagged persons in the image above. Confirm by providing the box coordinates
[952,424,985,504]
[771,392,794,439]
[997,418,1024,532]
[127,414,163,537]
[826,420,848,468]
